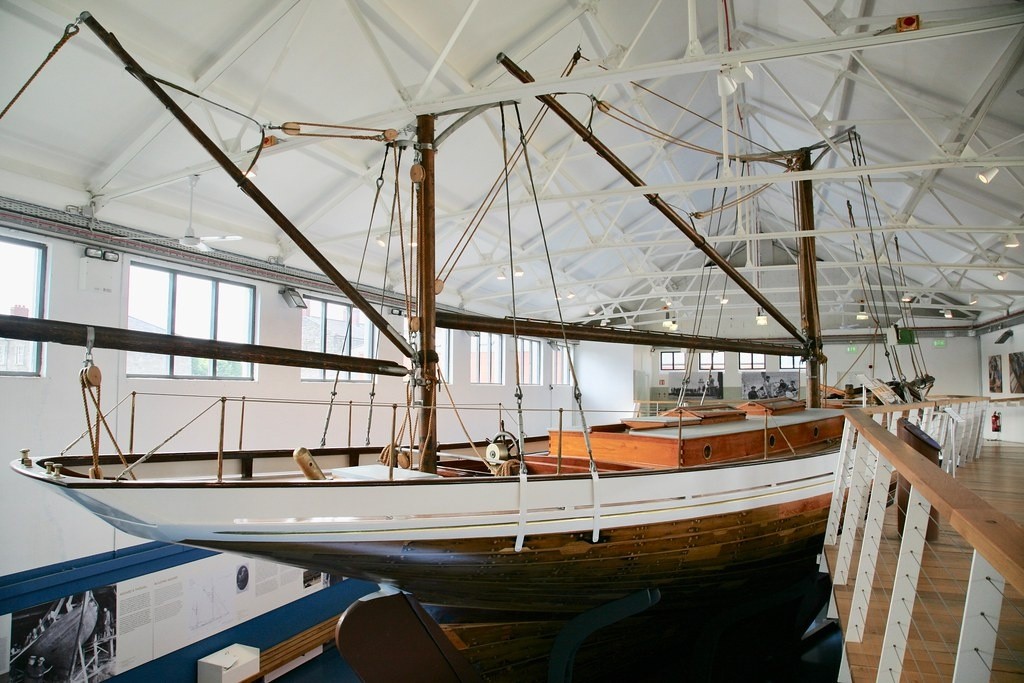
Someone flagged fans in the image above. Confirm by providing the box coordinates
[112,174,241,253]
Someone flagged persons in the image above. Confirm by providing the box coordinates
[747,376,798,399]
[709,375,714,387]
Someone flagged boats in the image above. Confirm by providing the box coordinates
[10,596,98,683]
[0,12,991,612]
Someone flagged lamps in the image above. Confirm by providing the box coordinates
[756,315,768,325]
[547,340,560,352]
[555,289,561,300]
[978,167,999,183]
[565,289,576,298]
[968,293,978,305]
[496,267,507,279]
[279,286,308,309]
[669,317,678,329]
[945,309,952,318]
[994,330,1013,344]
[390,309,404,315]
[662,312,672,326]
[240,160,258,176]
[1005,233,1019,247]
[377,232,388,247]
[717,64,753,96]
[513,266,524,276]
[856,300,869,320]
[996,271,1008,280]
[85,247,119,263]
[902,293,913,301]
[408,239,417,246]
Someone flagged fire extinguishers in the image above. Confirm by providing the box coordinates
[991,410,1001,432]
